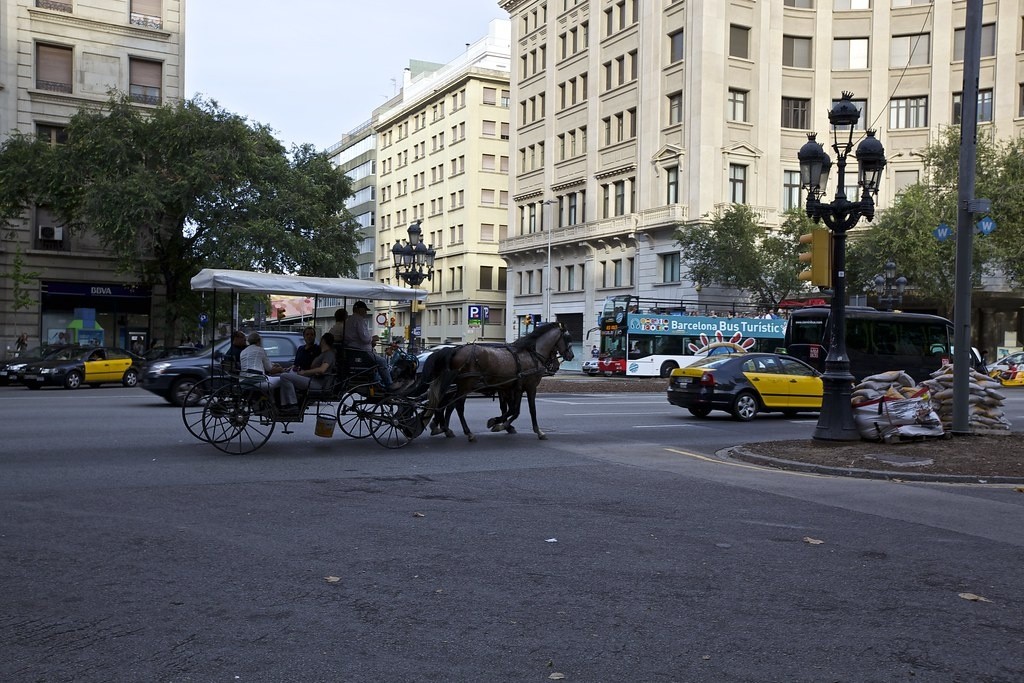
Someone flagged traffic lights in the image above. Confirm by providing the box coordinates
[277,308,285,320]
[526,315,532,325]
[403,325,410,340]
[391,312,396,327]
[412,285,426,313]
[797,229,829,286]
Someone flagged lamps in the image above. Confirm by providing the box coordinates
[542,200,557,205]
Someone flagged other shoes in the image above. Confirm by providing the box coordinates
[280,404,298,412]
[387,381,403,393]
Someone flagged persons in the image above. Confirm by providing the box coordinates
[178,337,203,349]
[59,332,67,344]
[591,345,599,358]
[132,336,144,356]
[15,333,28,357]
[1001,361,1018,380]
[330,301,403,390]
[630,345,641,354]
[613,305,779,324]
[227,327,337,411]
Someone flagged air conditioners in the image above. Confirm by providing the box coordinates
[38,225,63,241]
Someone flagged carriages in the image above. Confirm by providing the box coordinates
[181,268,576,455]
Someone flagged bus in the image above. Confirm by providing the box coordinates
[587,294,797,378]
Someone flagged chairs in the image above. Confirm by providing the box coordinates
[765,364,779,373]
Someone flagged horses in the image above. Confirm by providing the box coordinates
[422,320,575,442]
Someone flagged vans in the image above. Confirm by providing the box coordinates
[784,307,990,387]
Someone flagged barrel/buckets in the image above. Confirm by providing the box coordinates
[314,403,337,438]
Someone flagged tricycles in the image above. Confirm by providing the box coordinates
[989,370,1024,386]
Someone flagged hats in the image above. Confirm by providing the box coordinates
[355,301,371,312]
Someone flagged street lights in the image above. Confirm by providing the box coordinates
[390,225,436,355]
[874,262,907,311]
[797,91,886,443]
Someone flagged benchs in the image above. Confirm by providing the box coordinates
[205,354,265,401]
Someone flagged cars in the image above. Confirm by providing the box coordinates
[985,352,1024,372]
[20,346,147,390]
[139,331,321,407]
[581,354,604,376]
[0,345,80,390]
[666,352,823,421]
